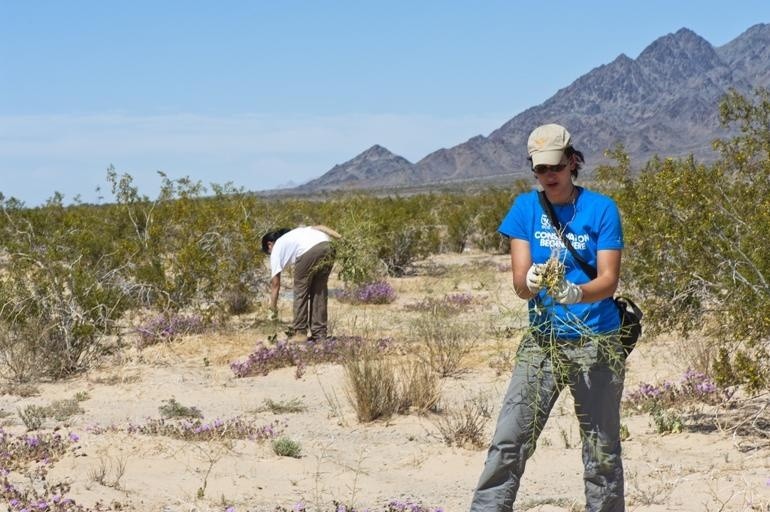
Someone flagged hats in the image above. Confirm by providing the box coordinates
[527,124,573,169]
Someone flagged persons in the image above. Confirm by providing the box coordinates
[261,225,341,343]
[470,123,625,512]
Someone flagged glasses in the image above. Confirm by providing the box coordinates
[531,158,572,173]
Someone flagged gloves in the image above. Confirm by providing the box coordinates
[270,313,279,321]
[554,281,583,306]
[525,264,549,295]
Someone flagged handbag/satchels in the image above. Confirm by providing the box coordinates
[614,296,641,360]
